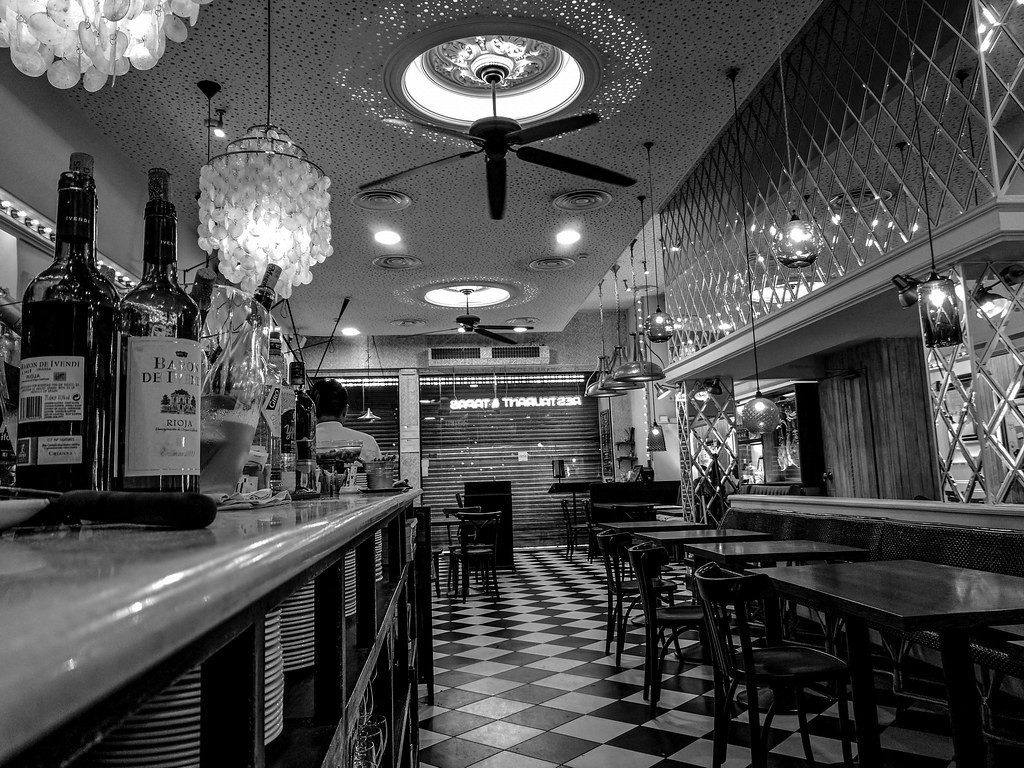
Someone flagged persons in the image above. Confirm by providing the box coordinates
[305,379,384,462]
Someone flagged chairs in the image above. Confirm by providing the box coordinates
[698,561,855,767]
[583,502,618,564]
[598,528,682,666]
[446,512,506,604]
[561,500,589,560]
[431,551,443,598]
[443,507,481,592]
[628,542,734,719]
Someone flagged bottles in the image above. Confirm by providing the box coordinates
[257,332,298,495]
[289,361,318,492]
[187,263,282,497]
[14,171,124,499]
[100,199,203,495]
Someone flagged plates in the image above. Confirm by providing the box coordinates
[345,549,356,617]
[0,498,50,532]
[281,578,316,672]
[80,607,285,767]
[376,529,383,582]
[358,488,404,493]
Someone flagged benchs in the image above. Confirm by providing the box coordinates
[680,507,1022,750]
[654,484,683,521]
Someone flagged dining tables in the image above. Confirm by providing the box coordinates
[635,529,774,609]
[431,519,477,587]
[683,544,870,665]
[597,521,707,584]
[744,558,1024,768]
[586,503,658,561]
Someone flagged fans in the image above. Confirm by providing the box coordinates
[420,290,535,346]
[359,53,637,219]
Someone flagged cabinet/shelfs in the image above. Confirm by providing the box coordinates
[463,481,515,571]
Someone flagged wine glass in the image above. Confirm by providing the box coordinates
[315,440,364,500]
[352,714,389,768]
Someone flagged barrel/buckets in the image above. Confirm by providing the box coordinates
[364,461,395,489]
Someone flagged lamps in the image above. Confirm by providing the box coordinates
[723,67,779,435]
[708,378,733,397]
[775,0,825,270]
[614,238,663,384]
[974,283,1008,320]
[642,141,674,343]
[584,281,627,399]
[889,274,922,310]
[900,1,962,348]
[694,382,708,401]
[638,195,666,452]
[214,107,226,139]
[599,263,644,391]
[0,0,213,93]
[198,1,332,299]
